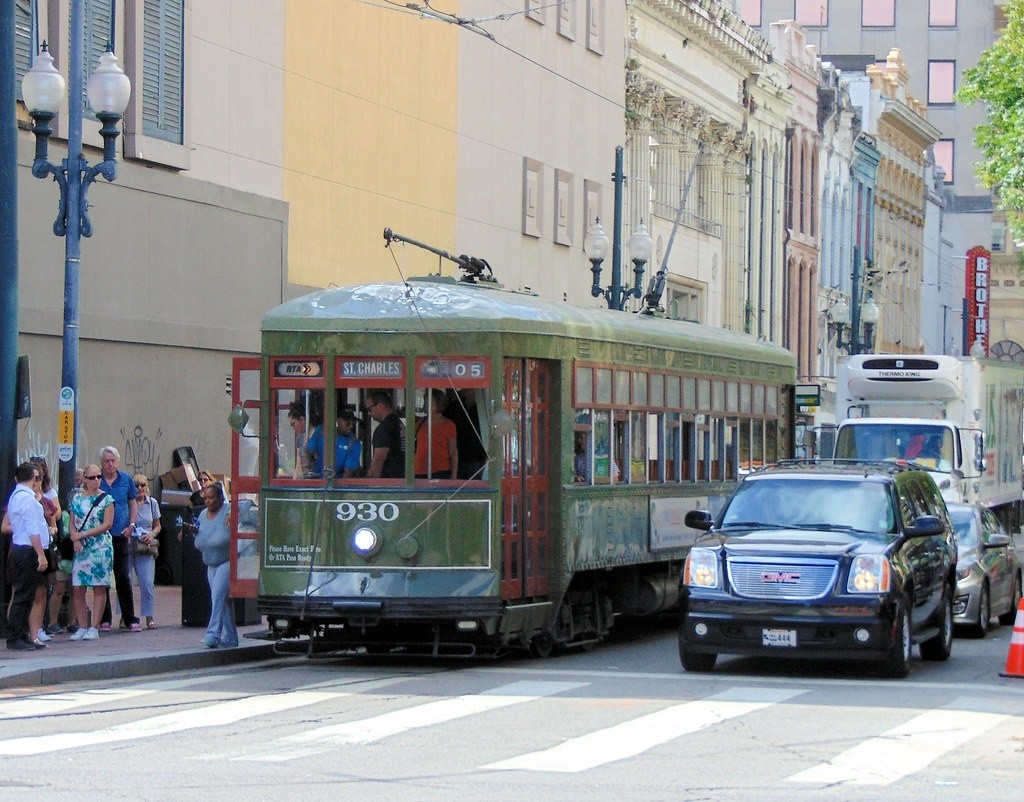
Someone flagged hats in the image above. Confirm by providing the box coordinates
[337,407,363,423]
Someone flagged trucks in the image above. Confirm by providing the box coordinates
[801,352,1024,537]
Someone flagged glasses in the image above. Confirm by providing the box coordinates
[291,418,299,426]
[365,402,383,412]
[135,483,146,487]
[30,457,46,465]
[35,476,42,481]
[84,475,102,480]
[199,479,211,483]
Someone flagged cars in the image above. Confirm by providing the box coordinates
[946,504,1024,638]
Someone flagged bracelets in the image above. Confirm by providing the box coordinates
[130,522,136,526]
[83,531,87,538]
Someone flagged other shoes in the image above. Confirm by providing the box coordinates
[36,628,51,642]
[130,623,142,631]
[201,638,218,648]
[99,623,110,632]
[34,639,50,648]
[119,619,127,629]
[7,639,36,651]
[146,620,157,628]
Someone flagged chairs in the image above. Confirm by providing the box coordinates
[615,459,732,481]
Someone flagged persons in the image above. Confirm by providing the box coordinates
[68,465,115,641]
[365,391,406,479]
[188,471,214,508]
[0,456,79,651]
[413,387,459,480]
[193,482,252,649]
[98,447,143,631]
[115,474,162,630]
[573,430,620,483]
[287,391,363,479]
[927,435,942,455]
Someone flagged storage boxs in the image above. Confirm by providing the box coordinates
[159,464,232,508]
[233,597,262,626]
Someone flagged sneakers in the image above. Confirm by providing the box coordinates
[44,623,63,634]
[69,628,87,640]
[82,627,99,640]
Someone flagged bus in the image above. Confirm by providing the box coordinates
[224,221,798,661]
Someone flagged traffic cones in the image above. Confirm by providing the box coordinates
[998,597,1024,678]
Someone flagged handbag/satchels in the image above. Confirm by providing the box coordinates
[133,535,159,555]
[60,535,74,560]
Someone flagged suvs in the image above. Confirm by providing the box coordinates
[677,457,959,681]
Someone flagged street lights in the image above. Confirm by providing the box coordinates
[21,36,131,516]
[584,143,653,311]
[831,291,881,357]
